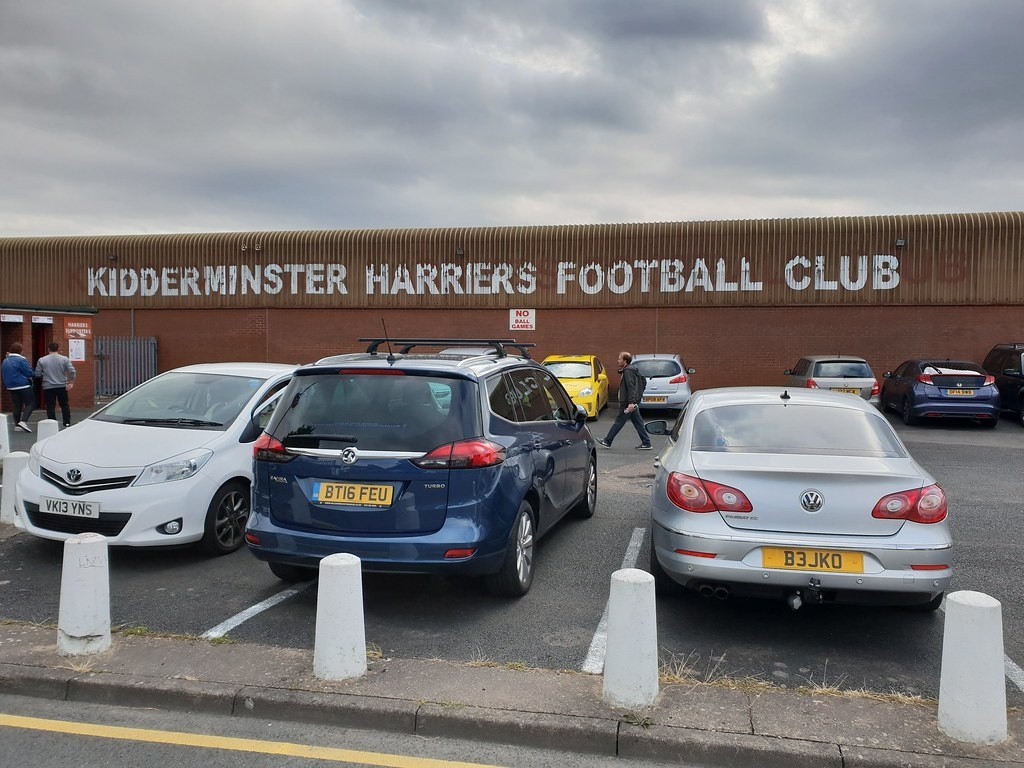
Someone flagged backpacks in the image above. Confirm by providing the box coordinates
[623,366,647,395]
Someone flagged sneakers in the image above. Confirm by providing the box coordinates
[14,427,25,431]
[595,436,611,449]
[635,443,652,449]
[18,420,32,432]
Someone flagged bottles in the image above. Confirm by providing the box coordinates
[624,404,638,414]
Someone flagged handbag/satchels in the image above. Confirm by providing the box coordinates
[29,377,33,386]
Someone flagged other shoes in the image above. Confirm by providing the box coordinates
[66,422,70,426]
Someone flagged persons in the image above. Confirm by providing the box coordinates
[1,342,35,433]
[595,351,653,450]
[35,342,76,428]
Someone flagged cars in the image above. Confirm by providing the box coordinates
[12,362,303,556]
[644,385,953,612]
[424,348,496,411]
[521,354,609,423]
[879,359,1002,428]
[617,353,696,417]
[782,354,884,413]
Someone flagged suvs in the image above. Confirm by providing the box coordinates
[983,342,1024,427]
[243,335,598,598]
